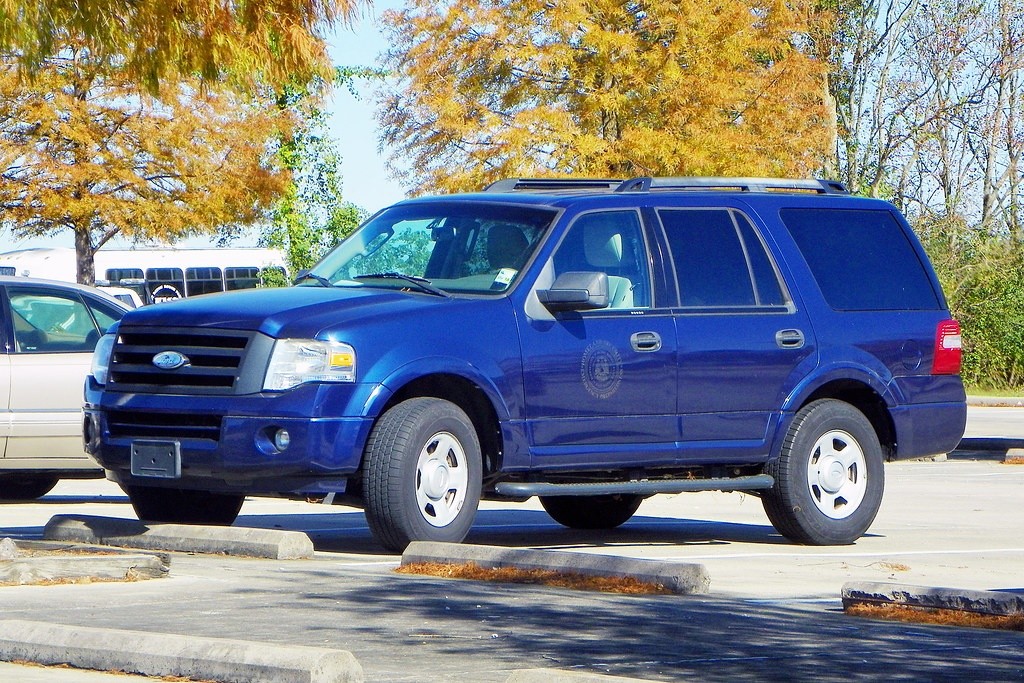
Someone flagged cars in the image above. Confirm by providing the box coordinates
[0,274,136,502]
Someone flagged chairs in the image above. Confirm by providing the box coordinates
[487,226,529,274]
[583,221,642,308]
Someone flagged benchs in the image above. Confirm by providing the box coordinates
[18,328,106,351]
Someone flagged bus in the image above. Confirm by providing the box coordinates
[29,247,292,307]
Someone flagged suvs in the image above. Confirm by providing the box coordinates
[11,286,144,344]
[81,176,967,556]
